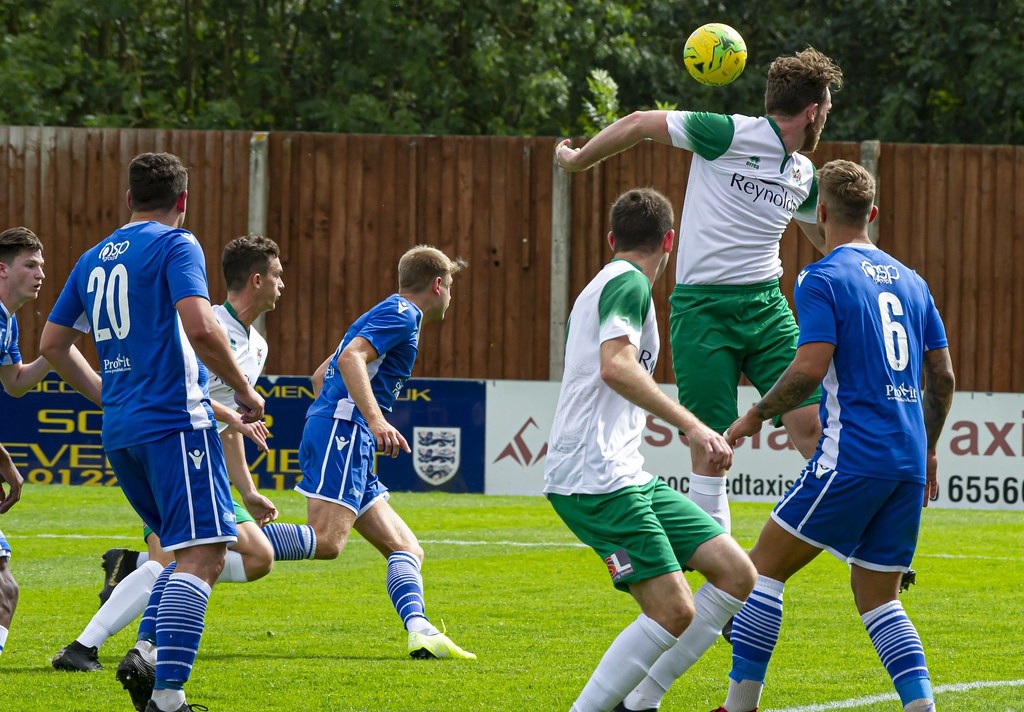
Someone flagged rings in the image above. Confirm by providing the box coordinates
[722,429,729,441]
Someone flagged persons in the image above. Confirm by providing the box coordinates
[50,234,286,677]
[719,156,958,710]
[550,46,844,545]
[40,148,270,712]
[235,245,481,662]
[546,184,758,712]
[0,223,53,662]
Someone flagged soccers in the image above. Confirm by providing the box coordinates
[683,23,747,86]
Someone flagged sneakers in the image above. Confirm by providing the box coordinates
[407,618,476,660]
[99,548,139,612]
[51,640,105,672]
[115,648,156,712]
[144,697,209,711]
